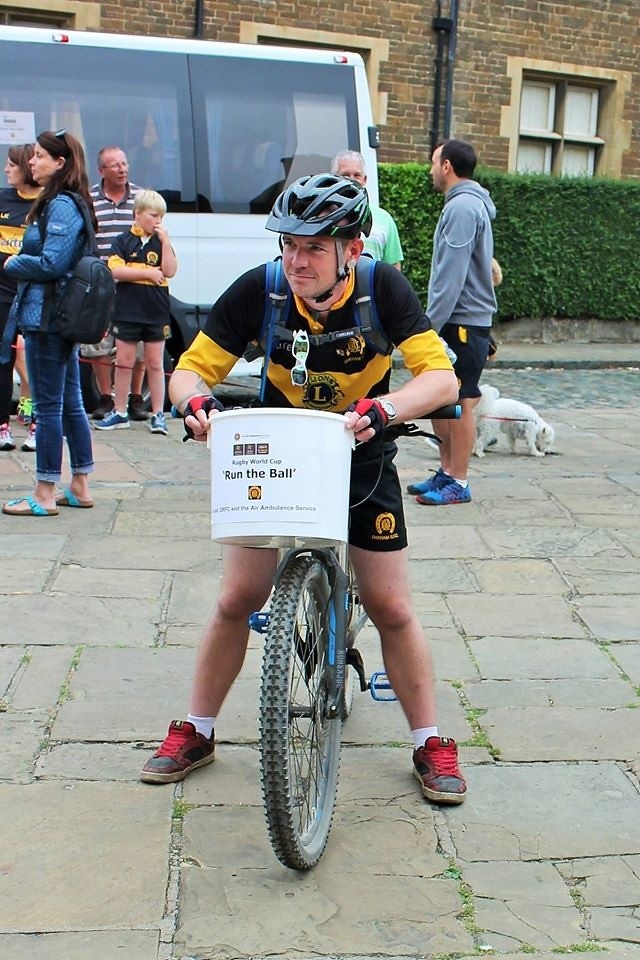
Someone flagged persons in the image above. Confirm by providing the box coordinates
[406,139,501,509]
[328,147,405,274]
[86,142,155,421]
[2,128,98,518]
[92,189,177,437]
[11,336,32,426]
[0,141,48,452]
[139,171,470,808]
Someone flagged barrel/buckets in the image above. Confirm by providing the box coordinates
[208,407,351,548]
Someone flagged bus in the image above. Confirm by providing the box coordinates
[2,20,387,428]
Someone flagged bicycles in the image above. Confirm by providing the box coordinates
[179,396,465,878]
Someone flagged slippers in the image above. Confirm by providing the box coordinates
[2,496,59,516]
[55,487,94,507]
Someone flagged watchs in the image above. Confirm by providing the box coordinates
[373,396,398,429]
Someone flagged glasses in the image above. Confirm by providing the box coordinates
[55,128,70,158]
[291,329,310,387]
[100,162,128,169]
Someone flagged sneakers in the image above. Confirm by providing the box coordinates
[21,422,37,451]
[16,397,34,425]
[416,480,471,505]
[140,720,215,783]
[0,422,16,450]
[406,467,456,495]
[94,408,130,430]
[149,411,168,435]
[412,736,467,803]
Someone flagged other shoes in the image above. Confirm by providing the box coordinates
[128,394,149,421]
[92,395,114,419]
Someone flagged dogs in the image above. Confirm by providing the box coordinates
[474,384,556,458]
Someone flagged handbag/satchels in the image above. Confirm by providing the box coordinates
[37,190,116,345]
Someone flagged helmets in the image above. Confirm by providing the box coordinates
[265,173,372,244]
